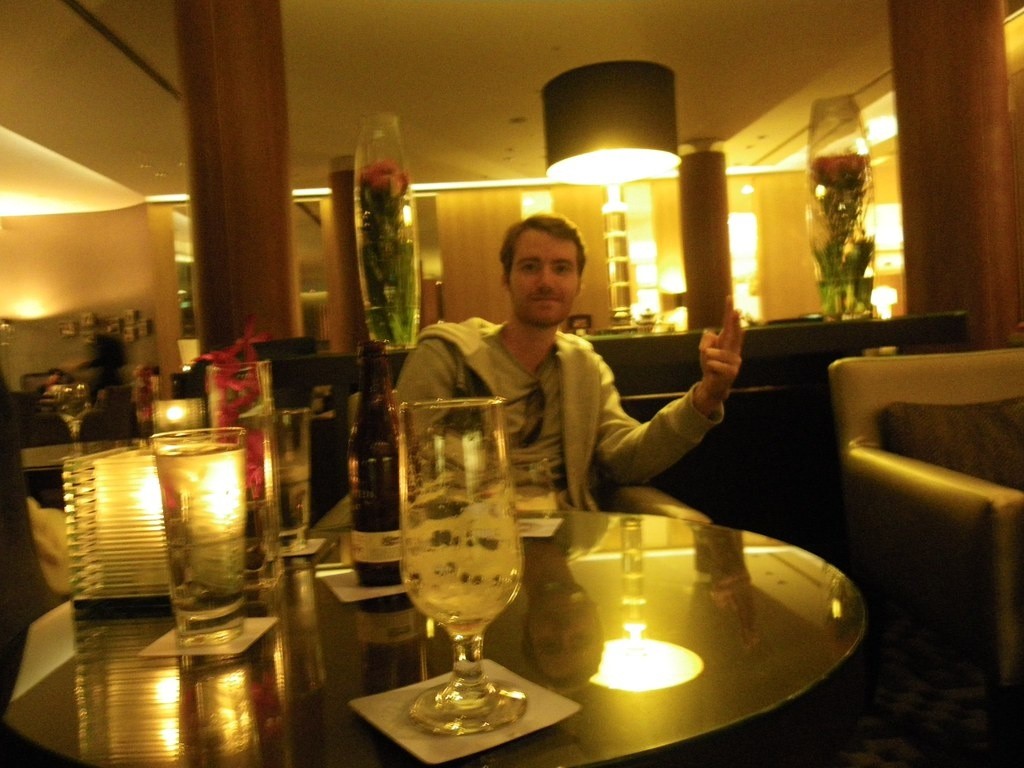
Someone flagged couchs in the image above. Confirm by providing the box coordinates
[828,348,1024,686]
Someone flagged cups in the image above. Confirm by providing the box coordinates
[512,457,558,521]
[149,426,246,647]
[191,657,260,758]
[205,360,279,589]
[271,407,312,553]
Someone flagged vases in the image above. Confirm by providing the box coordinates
[816,276,876,319]
[366,307,421,349]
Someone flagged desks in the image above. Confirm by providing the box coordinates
[0,509,868,768]
[21,439,146,485]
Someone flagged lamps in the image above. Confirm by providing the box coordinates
[62,447,173,621]
[542,60,682,188]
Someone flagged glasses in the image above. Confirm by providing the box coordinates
[518,380,545,449]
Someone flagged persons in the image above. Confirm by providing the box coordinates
[30,368,67,395]
[78,332,127,408]
[399,212,744,513]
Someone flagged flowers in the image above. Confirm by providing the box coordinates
[185,317,278,534]
[807,94,876,275]
[352,110,421,307]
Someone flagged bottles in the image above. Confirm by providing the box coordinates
[349,340,400,586]
[358,593,427,767]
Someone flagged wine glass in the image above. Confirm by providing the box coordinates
[400,397,525,735]
[55,383,92,461]
